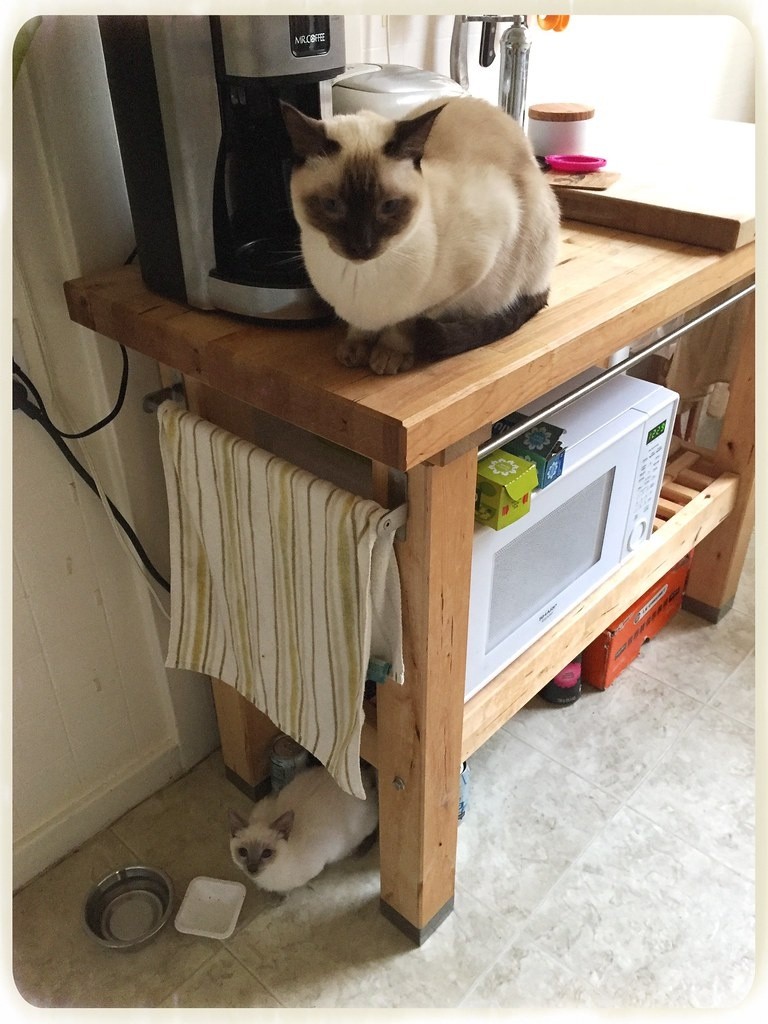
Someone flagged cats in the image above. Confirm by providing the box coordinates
[227,762,381,894]
[281,96,562,377]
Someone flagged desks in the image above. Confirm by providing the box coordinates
[61,216,757,947]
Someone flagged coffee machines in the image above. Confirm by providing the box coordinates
[97,15,350,326]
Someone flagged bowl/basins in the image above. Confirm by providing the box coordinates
[81,863,175,951]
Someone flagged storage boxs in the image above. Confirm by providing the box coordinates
[492,410,565,489]
[577,547,694,692]
[475,448,539,531]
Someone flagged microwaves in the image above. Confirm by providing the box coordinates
[460,366,681,705]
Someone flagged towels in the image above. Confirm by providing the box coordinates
[156,400,404,800]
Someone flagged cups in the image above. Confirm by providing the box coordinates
[527,117,594,161]
